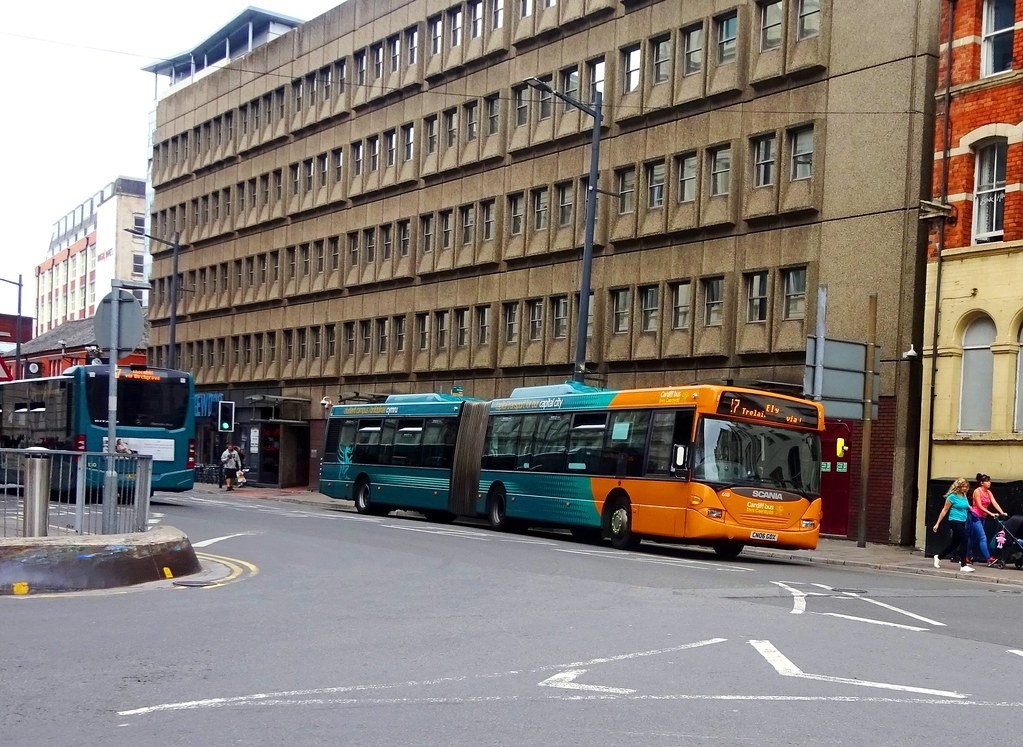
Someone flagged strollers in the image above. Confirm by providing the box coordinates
[995,512,1023,568]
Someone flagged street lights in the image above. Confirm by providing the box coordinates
[101,278,152,535]
[521,78,609,388]
[124,225,186,371]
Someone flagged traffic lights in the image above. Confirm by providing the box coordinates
[217,400,236,433]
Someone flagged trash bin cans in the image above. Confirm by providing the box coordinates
[23,446,51,537]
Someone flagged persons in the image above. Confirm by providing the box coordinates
[222,443,245,491]
[116,439,138,459]
[933,473,1007,572]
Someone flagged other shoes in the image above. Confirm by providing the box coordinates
[226,487,234,491]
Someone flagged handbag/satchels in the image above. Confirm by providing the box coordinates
[990,525,1005,550]
[236,470,247,483]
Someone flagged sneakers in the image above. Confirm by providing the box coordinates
[987,557,998,567]
[934,554,941,569]
[959,557,972,566]
[960,566,975,572]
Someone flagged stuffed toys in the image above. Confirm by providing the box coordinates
[996,531,1006,549]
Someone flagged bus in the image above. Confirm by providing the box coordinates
[0,361,197,502]
[316,373,855,559]
